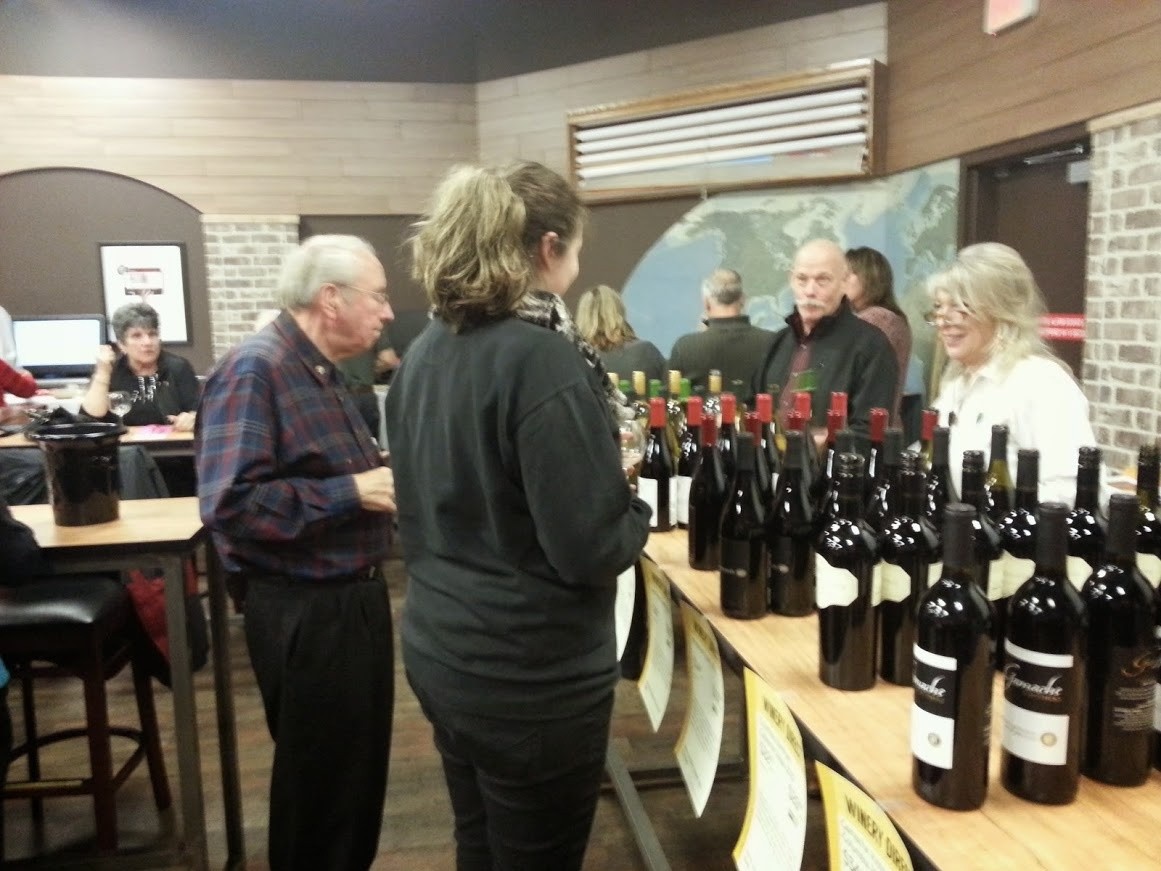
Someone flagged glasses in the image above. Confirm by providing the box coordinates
[924,311,967,327]
[333,279,391,302]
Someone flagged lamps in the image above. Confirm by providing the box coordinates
[566,58,885,206]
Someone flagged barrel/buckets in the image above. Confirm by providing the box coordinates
[24,423,128,527]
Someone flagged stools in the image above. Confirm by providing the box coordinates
[0,587,175,851]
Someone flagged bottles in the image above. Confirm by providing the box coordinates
[607,362,1161,812]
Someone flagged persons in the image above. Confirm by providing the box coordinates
[385,158,655,871]
[0,304,202,591]
[745,237,912,453]
[906,243,1109,523]
[193,235,400,871]
[574,269,778,403]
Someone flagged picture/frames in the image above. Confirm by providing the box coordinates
[96,240,194,351]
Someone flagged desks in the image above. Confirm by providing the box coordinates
[0,506,244,871]
[0,420,245,623]
[606,527,1161,871]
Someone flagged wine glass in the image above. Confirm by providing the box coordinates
[108,389,132,427]
